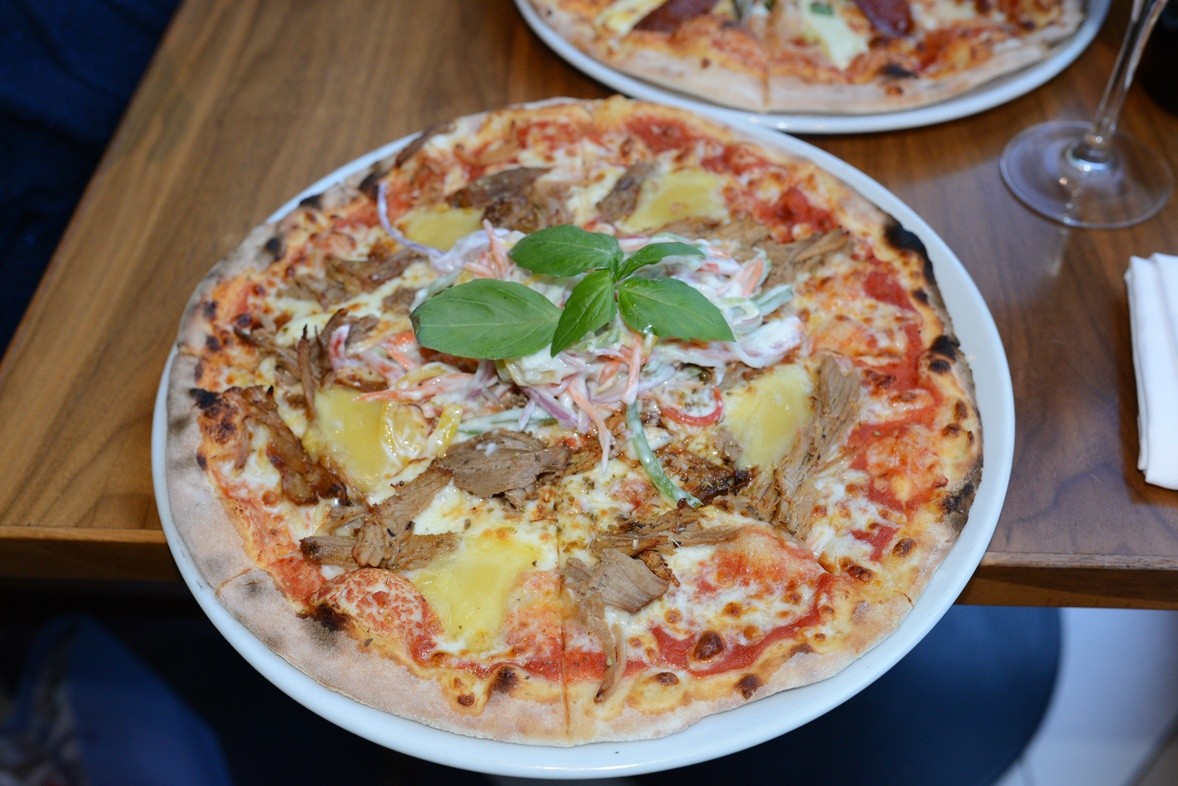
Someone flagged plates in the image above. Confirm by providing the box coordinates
[514,0,1107,133]
[151,98,1015,781]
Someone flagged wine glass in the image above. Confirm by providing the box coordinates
[997,0,1174,228]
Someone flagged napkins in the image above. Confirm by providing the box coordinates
[1124,252,1178,490]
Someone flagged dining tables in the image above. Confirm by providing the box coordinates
[0,0,1178,611]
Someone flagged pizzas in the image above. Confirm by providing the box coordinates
[161,93,986,748]
[530,1,1087,115]
